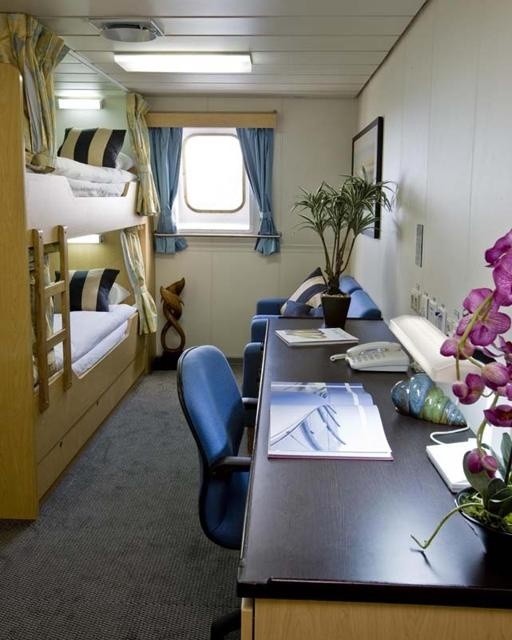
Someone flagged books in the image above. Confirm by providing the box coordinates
[268,380,394,461]
[275,328,359,347]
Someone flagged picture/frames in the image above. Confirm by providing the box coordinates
[350,116,383,239]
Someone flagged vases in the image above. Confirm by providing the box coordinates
[454,486,511,555]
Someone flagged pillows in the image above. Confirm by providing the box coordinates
[280,267,326,317]
[56,128,135,169]
[52,268,132,312]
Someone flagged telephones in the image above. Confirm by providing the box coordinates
[344,341,410,373]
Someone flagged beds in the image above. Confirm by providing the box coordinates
[1,65,156,521]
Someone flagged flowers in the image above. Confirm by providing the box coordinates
[411,229,512,549]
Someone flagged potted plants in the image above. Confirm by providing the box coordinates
[290,166,399,329]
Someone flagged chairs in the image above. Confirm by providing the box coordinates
[243,275,381,398]
[176,344,259,639]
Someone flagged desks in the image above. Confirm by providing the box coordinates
[238,319,511,640]
